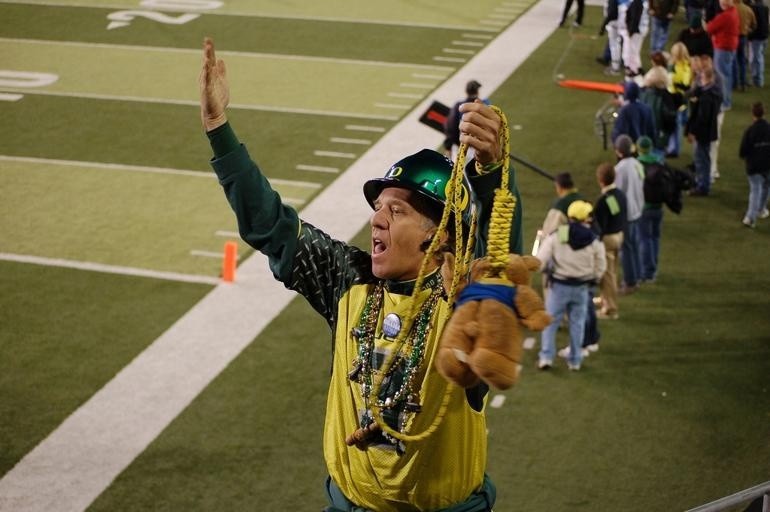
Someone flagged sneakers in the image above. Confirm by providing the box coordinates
[557,347,589,357]
[759,208,770,219]
[743,216,756,228]
[592,297,602,304]
[584,344,599,352]
[567,361,581,370]
[595,308,619,319]
[538,359,552,369]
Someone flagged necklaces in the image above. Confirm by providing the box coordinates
[344,276,444,455]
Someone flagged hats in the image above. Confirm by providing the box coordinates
[567,200,593,219]
[637,136,651,152]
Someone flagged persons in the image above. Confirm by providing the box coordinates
[438,77,489,161]
[529,135,665,371]
[560,0,769,228]
[197,36,517,510]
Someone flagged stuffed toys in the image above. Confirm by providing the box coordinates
[436,251,551,390]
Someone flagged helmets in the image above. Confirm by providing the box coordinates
[363,150,470,230]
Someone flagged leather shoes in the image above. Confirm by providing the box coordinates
[687,188,707,196]
[619,287,635,294]
